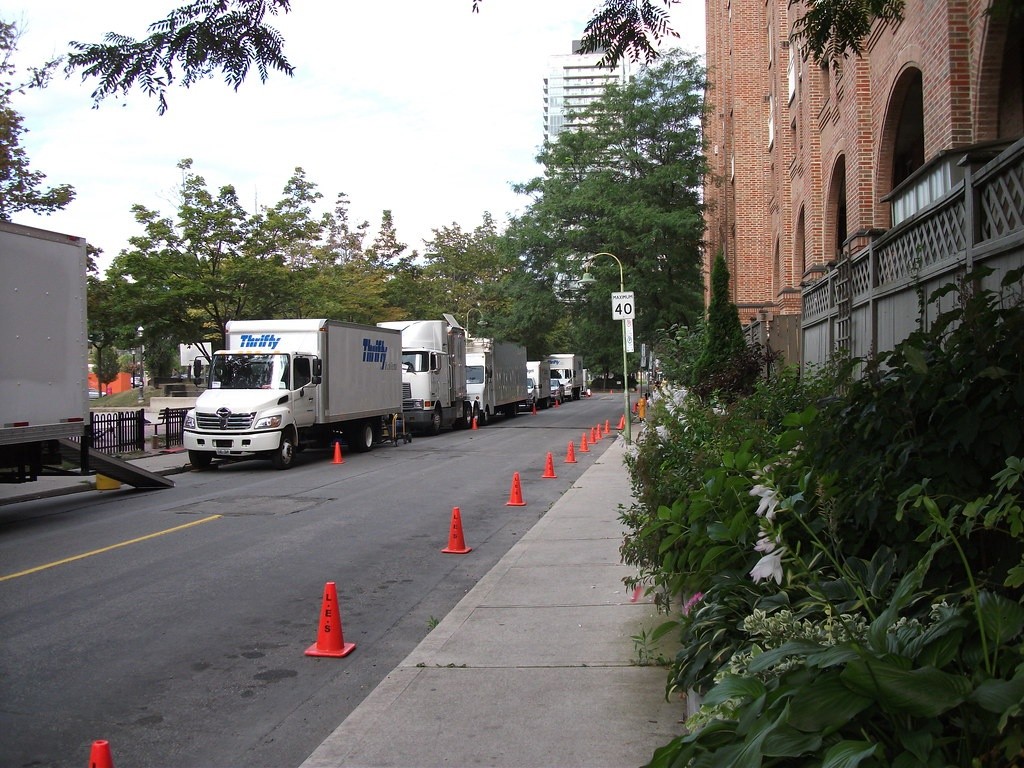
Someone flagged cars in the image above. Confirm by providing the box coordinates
[88,388,106,399]
[550,379,565,406]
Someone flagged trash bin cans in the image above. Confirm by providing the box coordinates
[106,387,112,396]
[638,397,645,420]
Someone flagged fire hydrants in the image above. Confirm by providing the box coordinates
[637,397,648,422]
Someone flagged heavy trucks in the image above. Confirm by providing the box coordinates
[376,314,481,436]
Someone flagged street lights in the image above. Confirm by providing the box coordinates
[137,326,145,403]
[577,253,631,446]
[131,347,137,389]
[467,308,488,338]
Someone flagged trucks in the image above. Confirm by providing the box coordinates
[182,319,404,470]
[466,337,551,426]
[544,354,588,402]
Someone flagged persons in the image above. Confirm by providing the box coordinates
[171,369,181,378]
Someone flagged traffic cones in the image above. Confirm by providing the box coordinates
[471,418,479,430]
[506,472,527,506]
[304,581,356,657]
[88,740,113,768]
[330,441,346,464]
[564,403,637,463]
[532,400,559,415]
[441,507,472,554]
[541,452,558,478]
